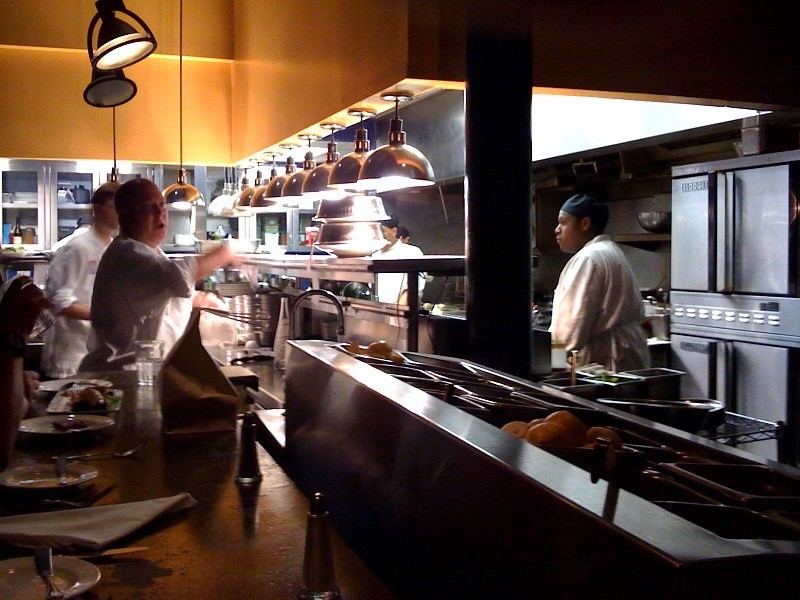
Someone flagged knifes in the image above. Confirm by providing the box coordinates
[36,544,64,600]
[55,454,68,485]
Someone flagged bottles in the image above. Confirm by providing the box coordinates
[296,492,341,600]
[236,411,263,485]
[274,297,291,371]
[8,225,37,243]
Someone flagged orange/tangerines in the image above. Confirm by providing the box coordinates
[502,411,618,451]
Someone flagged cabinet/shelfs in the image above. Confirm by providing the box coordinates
[0,164,326,252]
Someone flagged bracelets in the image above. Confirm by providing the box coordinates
[0,329,28,356]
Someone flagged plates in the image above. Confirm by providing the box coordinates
[47,386,124,413]
[37,379,113,391]
[0,464,99,488]
[0,554,101,600]
[17,414,114,432]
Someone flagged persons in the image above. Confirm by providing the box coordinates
[371,214,429,306]
[548,194,652,374]
[0,177,255,473]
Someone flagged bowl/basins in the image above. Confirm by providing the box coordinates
[200,238,260,254]
[636,211,672,234]
[219,282,252,296]
[175,234,196,246]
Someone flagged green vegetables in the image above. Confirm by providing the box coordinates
[101,395,122,403]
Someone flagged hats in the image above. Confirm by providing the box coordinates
[561,193,610,231]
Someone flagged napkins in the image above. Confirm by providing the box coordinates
[0,490,197,555]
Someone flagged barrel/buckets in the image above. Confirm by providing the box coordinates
[230,294,283,346]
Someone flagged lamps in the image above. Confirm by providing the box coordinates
[94,107,122,193]
[208,89,438,218]
[86,0,157,72]
[83,67,138,109]
[160,0,206,211]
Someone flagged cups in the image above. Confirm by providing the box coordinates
[134,340,164,386]
[6,264,49,290]
[1,272,57,340]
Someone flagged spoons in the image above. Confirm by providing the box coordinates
[52,443,144,460]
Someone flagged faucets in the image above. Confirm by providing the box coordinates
[288,288,346,340]
